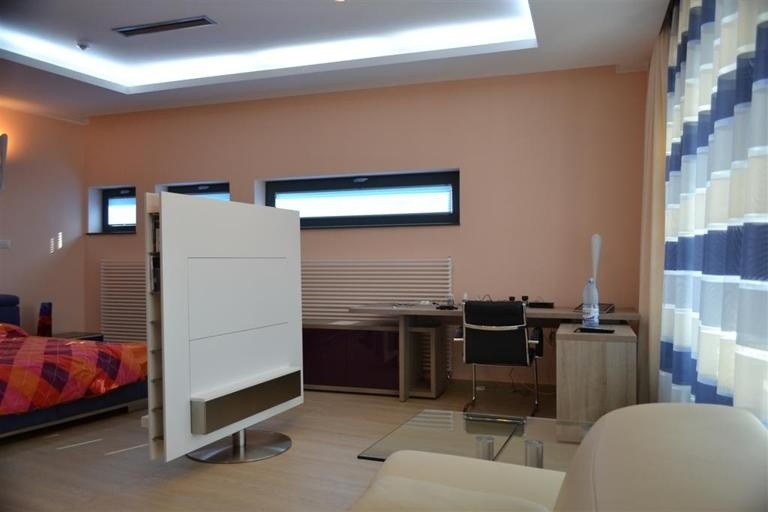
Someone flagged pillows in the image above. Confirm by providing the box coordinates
[0,321,28,339]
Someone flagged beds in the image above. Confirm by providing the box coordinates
[1,291,147,440]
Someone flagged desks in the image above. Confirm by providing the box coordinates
[355,409,596,474]
[348,302,639,402]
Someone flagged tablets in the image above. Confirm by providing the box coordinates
[574,302,614,313]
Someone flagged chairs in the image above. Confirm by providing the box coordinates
[350,402,768,512]
[453,297,543,426]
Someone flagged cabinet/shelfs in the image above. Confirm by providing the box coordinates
[303,317,446,398]
[551,323,639,444]
